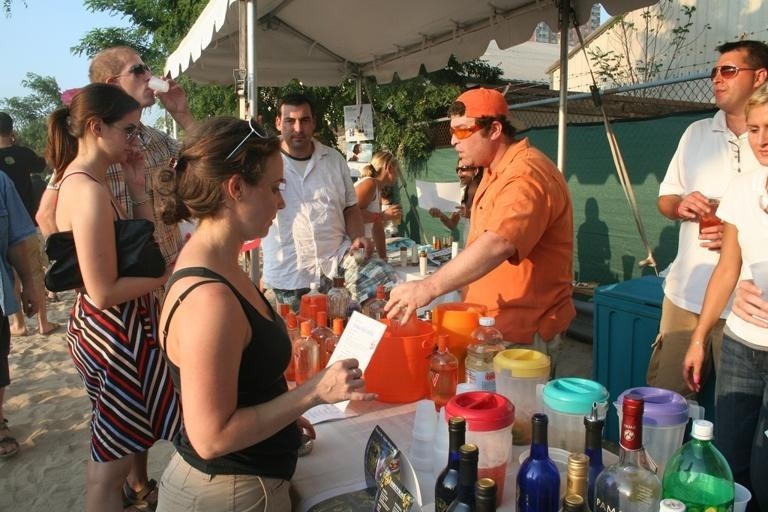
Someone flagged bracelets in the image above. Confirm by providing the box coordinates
[689,336,707,350]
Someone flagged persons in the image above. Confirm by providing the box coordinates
[384,85,575,384]
[681,87,768,511]
[35,45,200,511]
[1,110,62,458]
[640,41,768,404]
[158,116,378,512]
[730,274,768,330]
[426,155,483,249]
[261,95,407,310]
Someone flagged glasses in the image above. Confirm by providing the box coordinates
[224,118,270,158]
[107,123,136,143]
[448,124,486,140]
[111,64,150,81]
[709,64,759,82]
[456,165,477,173]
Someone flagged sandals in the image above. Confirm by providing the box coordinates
[121,478,160,504]
[1,418,19,458]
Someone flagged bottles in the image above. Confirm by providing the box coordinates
[390,236,453,275]
[411,398,449,494]
[434,416,498,512]
[578,396,736,512]
[463,314,504,393]
[277,274,413,394]
[516,411,590,511]
[428,335,458,404]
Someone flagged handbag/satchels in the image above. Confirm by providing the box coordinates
[44,219,166,293]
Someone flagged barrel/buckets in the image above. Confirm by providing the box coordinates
[365,318,435,404]
[433,302,501,380]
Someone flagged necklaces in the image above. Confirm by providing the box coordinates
[757,179,768,214]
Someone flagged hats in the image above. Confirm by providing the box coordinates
[449,88,527,129]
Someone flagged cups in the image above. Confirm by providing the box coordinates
[695,196,720,235]
[446,392,515,505]
[148,75,169,95]
[391,203,402,225]
[750,261,768,303]
[728,482,753,511]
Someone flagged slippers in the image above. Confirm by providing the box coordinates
[38,321,60,335]
[10,327,28,336]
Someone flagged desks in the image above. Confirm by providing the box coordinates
[279,306,621,511]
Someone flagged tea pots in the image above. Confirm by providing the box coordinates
[613,386,704,472]
[534,378,610,459]
[492,348,551,446]
